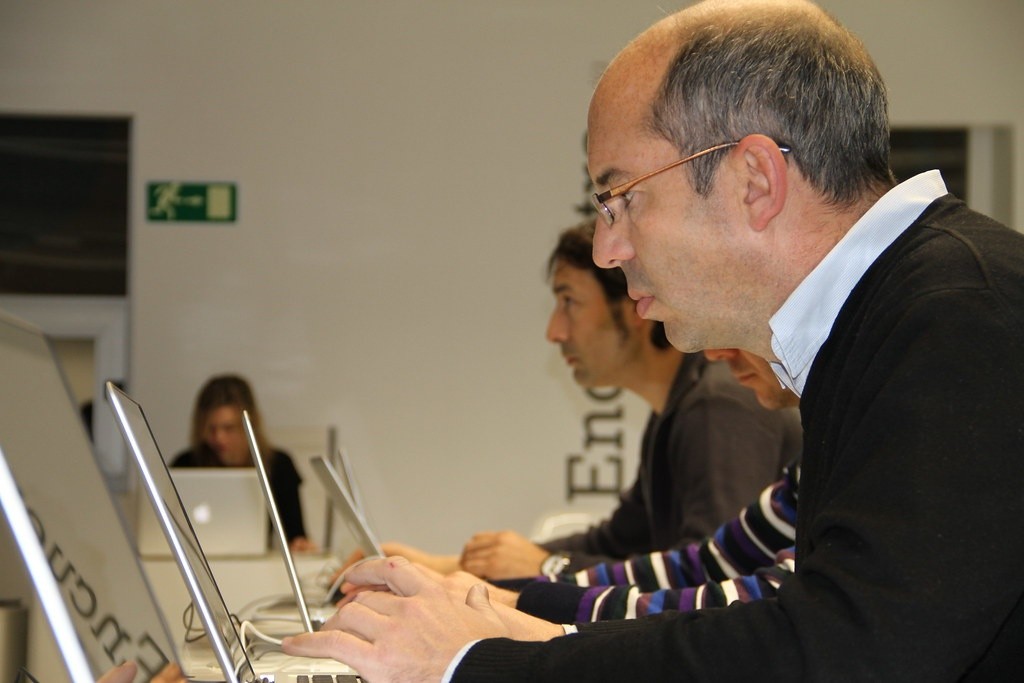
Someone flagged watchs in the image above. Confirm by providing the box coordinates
[539,549,571,584]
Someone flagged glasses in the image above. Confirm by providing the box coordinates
[590,140,792,229]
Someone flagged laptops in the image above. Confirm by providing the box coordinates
[0,310,391,683]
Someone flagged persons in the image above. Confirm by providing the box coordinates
[169,373,316,550]
[95,660,191,683]
[327,215,804,592]
[437,348,799,623]
[281,0,1024,683]
